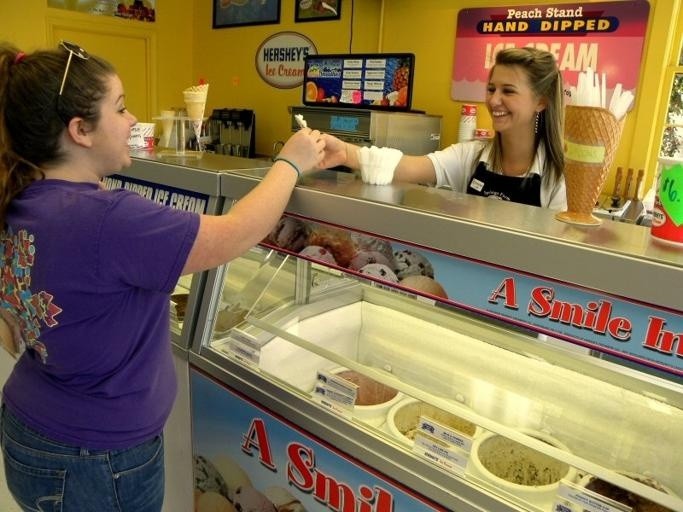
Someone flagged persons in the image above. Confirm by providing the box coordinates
[1,36,328,512]
[303,45,574,213]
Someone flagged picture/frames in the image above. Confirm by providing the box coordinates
[212,0,281,30]
[294,0,341,22]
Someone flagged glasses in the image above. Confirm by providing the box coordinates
[55,38,91,114]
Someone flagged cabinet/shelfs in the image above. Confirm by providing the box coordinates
[97,147,310,511]
[189,170,682,511]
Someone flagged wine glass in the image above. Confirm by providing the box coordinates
[555,102,629,227]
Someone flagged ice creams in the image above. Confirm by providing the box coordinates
[193,447,305,512]
[270,216,448,300]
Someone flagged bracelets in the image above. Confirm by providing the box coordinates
[272,153,301,177]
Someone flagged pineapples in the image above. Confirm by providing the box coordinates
[392,67,409,88]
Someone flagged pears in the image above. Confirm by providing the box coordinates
[373,100,381,105]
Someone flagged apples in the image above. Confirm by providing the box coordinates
[317,88,326,101]
[381,99,389,106]
